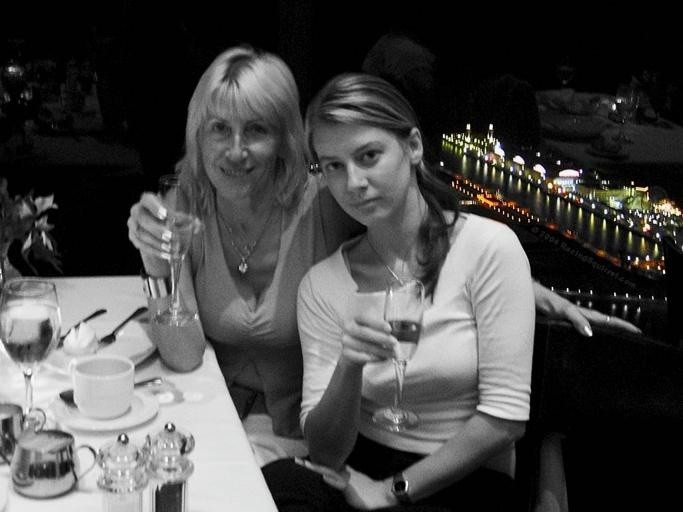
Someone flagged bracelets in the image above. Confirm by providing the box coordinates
[141,271,174,300]
[392,472,413,508]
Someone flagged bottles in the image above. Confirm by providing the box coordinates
[97,422,195,511]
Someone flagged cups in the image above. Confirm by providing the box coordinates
[0,402,96,501]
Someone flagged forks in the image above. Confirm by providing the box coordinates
[95,306,148,345]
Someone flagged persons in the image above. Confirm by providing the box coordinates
[126,48,645,466]
[259,86,644,511]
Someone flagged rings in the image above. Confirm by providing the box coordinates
[606,316,610,327]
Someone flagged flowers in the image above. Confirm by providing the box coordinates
[0,178,63,274]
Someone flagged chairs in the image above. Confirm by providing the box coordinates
[521,317,683,511]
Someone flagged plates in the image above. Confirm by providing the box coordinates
[43,316,158,432]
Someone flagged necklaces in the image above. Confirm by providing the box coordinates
[361,235,421,433]
[211,201,276,276]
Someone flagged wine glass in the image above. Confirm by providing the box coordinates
[1,277,63,412]
[372,278,426,432]
[153,172,200,331]
